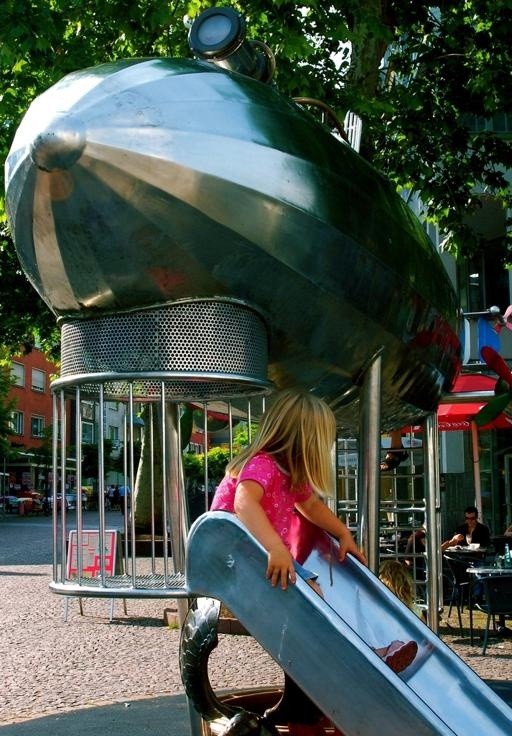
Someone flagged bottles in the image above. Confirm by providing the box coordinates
[503,542,511,569]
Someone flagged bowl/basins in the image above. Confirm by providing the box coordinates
[469,542,480,550]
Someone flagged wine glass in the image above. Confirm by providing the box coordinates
[465,534,474,549]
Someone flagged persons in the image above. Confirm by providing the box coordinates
[380,430,409,471]
[108,484,130,516]
[378,506,511,610]
[212,392,417,674]
[32,494,49,516]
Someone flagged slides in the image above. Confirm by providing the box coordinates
[185,510,512,735]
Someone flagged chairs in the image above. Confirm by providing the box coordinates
[423,545,512,655]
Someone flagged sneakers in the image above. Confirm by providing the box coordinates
[381,640,417,674]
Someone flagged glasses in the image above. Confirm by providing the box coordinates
[464,516,476,520]
